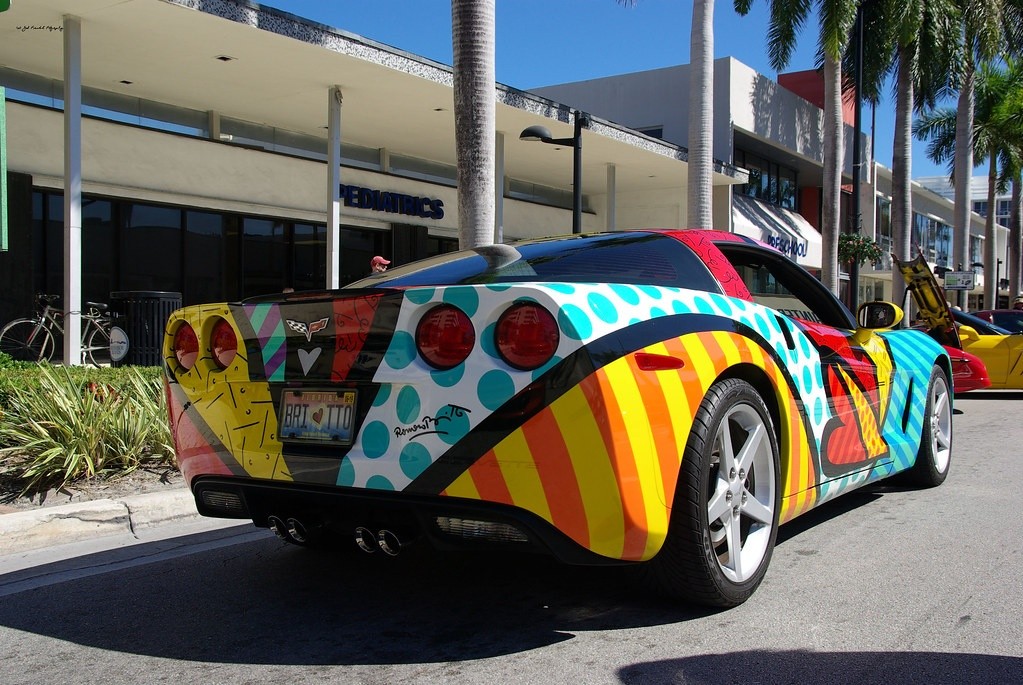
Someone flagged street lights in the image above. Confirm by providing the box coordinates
[519,110,588,233]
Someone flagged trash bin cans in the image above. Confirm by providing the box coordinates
[109,291,183,368]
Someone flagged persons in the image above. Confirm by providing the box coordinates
[371,256,391,273]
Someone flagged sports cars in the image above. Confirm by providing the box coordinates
[161,227,954,609]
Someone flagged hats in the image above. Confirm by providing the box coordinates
[371,256,390,266]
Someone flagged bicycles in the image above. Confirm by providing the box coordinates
[0,293,110,370]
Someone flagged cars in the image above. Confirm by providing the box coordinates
[915,307,1023,390]
[969,310,1023,334]
[885,243,992,395]
